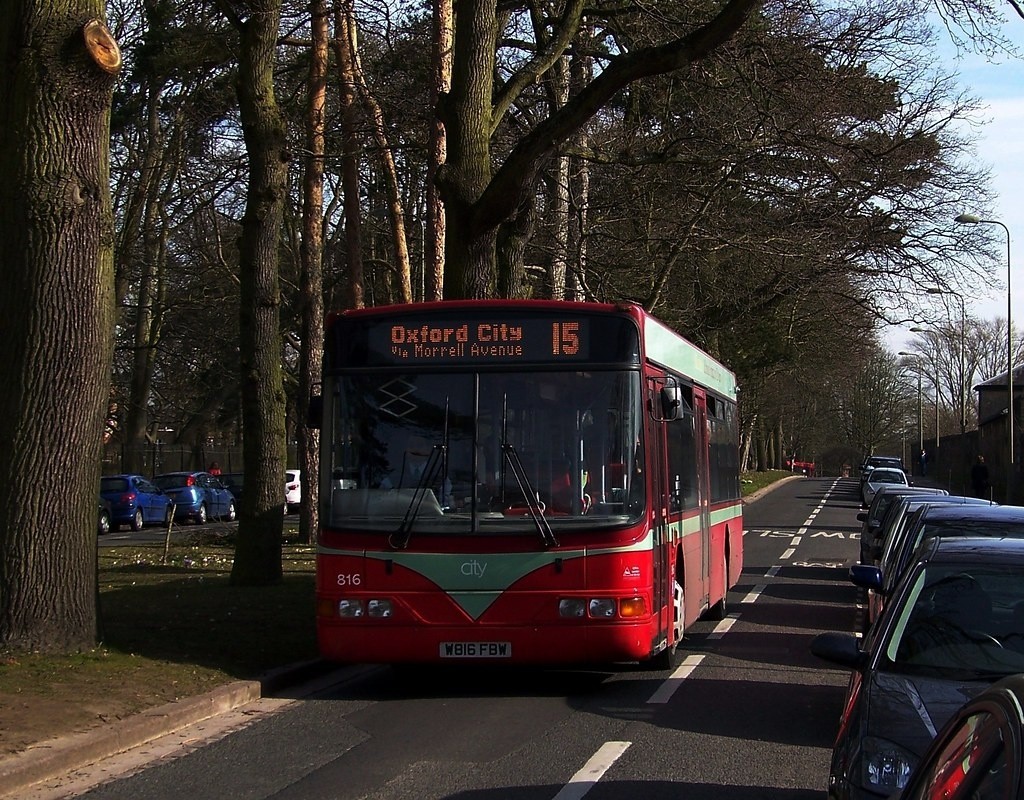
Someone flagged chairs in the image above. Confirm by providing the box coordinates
[487,446,638,516]
[916,574,993,640]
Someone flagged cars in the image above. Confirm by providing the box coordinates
[857,455,914,505]
[96,470,302,534]
[826,487,1024,800]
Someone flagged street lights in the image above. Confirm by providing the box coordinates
[926,287,966,435]
[910,327,940,470]
[897,351,924,476]
[954,214,1014,462]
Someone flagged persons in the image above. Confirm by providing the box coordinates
[377,427,463,508]
[207,461,222,475]
[972,455,989,497]
[916,449,929,477]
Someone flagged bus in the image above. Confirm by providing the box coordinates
[313,299,743,669]
